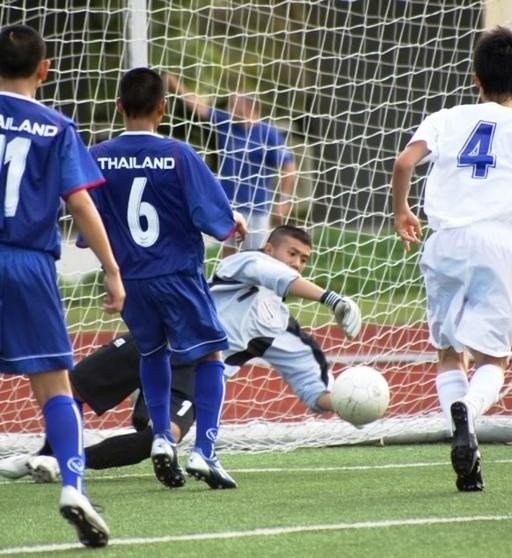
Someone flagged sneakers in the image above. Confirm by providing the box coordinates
[450,396,484,491]
[0,447,61,483]
[185,445,238,489]
[58,484,110,547]
[151,432,186,487]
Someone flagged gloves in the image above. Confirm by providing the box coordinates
[320,289,363,340]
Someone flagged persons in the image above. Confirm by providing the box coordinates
[0,225,390,483]
[389,26,512,491]
[167,75,296,261]
[76,67,247,488]
[0,23,126,549]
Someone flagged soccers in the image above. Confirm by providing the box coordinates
[330,366,390,423]
